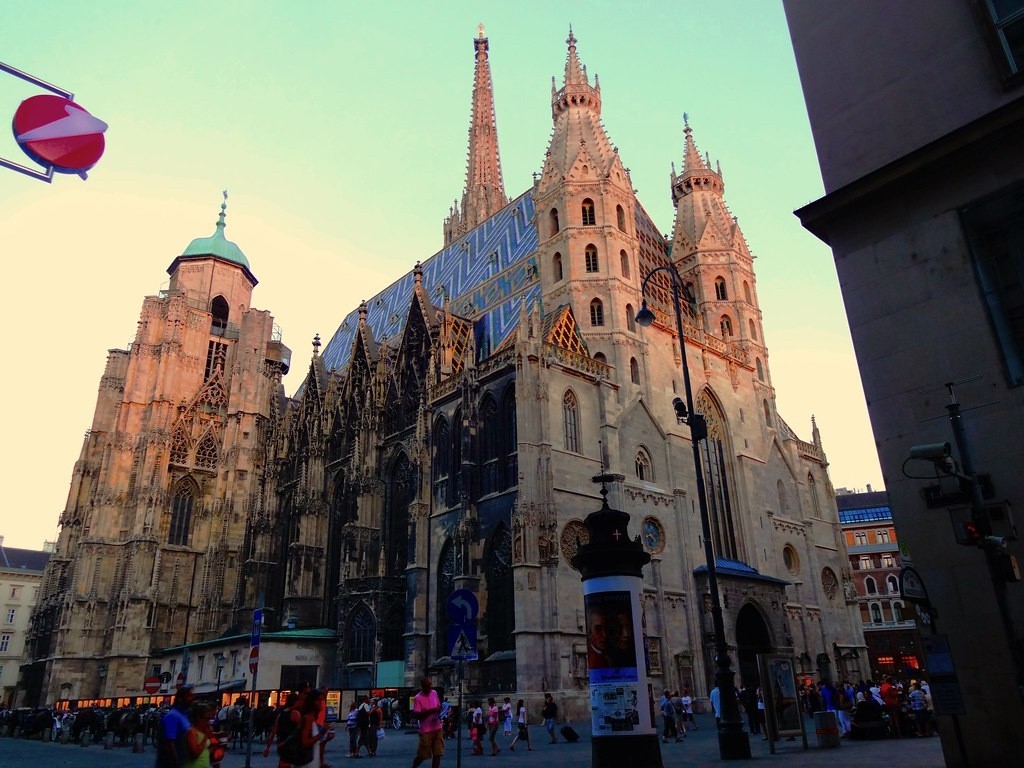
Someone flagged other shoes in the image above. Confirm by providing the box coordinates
[549,740,556,744]
[489,753,496,756]
[676,738,683,742]
[662,740,669,743]
[497,749,500,752]
[510,744,515,751]
[761,737,768,741]
[345,753,376,758]
[786,738,795,741]
[528,746,535,750]
[684,732,687,737]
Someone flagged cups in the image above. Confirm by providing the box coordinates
[326,723,335,733]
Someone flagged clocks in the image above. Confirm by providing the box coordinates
[643,519,662,550]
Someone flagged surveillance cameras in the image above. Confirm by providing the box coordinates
[910,443,952,460]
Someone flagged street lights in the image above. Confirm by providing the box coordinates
[215,654,224,709]
[637,260,753,763]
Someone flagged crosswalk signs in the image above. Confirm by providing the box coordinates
[253,611,260,646]
[450,625,477,663]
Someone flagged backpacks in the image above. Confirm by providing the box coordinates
[370,711,380,725]
[355,704,369,728]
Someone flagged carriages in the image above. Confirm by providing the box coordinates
[5,696,284,748]
[371,686,445,730]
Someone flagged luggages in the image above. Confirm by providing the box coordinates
[556,722,580,742]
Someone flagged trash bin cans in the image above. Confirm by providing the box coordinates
[814,711,840,748]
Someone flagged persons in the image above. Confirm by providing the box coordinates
[345,695,383,758]
[586,607,637,669]
[467,697,512,756]
[155,685,233,768]
[412,678,445,768]
[660,688,698,743]
[540,693,560,745]
[509,699,536,752]
[263,681,335,768]
[439,697,455,740]
[710,674,939,741]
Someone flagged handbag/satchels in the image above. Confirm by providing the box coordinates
[376,728,387,740]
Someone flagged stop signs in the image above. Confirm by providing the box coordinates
[249,647,259,673]
[175,673,183,690]
[144,676,162,694]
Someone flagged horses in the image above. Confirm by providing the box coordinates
[0,705,275,749]
[217,704,244,748]
